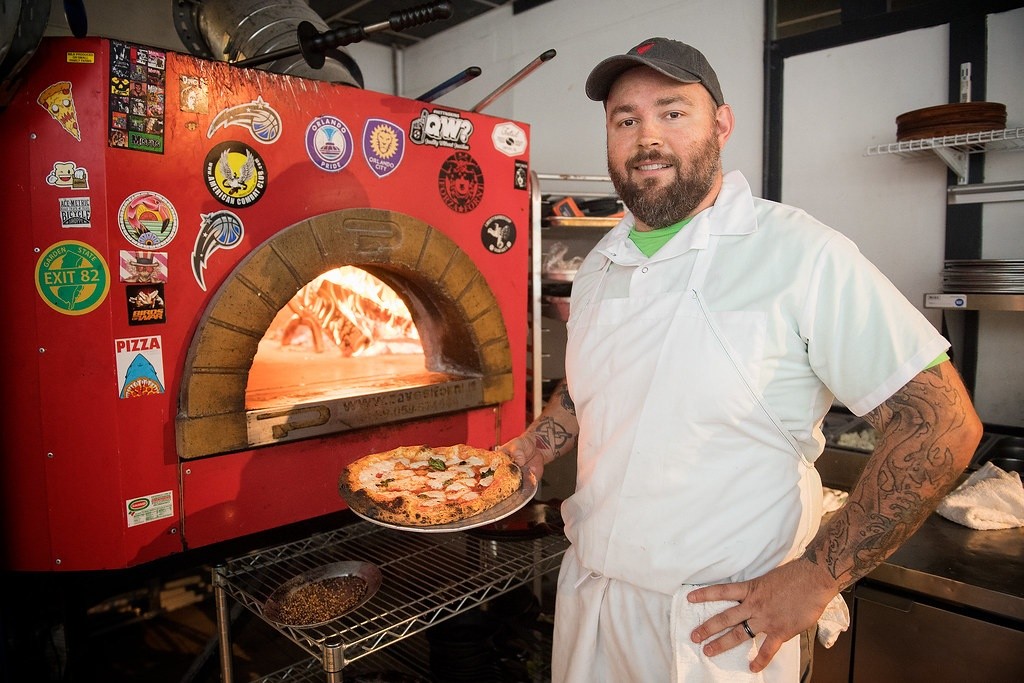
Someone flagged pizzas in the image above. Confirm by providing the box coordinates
[338,443,523,526]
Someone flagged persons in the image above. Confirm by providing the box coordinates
[491,37,983,683]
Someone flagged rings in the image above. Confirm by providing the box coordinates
[742,620,756,638]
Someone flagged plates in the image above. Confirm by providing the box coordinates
[346,465,538,533]
[263,561,382,629]
[896,102,1008,142]
[939,259,1024,293]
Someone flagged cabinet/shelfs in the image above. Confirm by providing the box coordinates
[761,0,1024,490]
[810,590,1024,683]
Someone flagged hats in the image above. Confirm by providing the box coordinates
[584,38,726,107]
